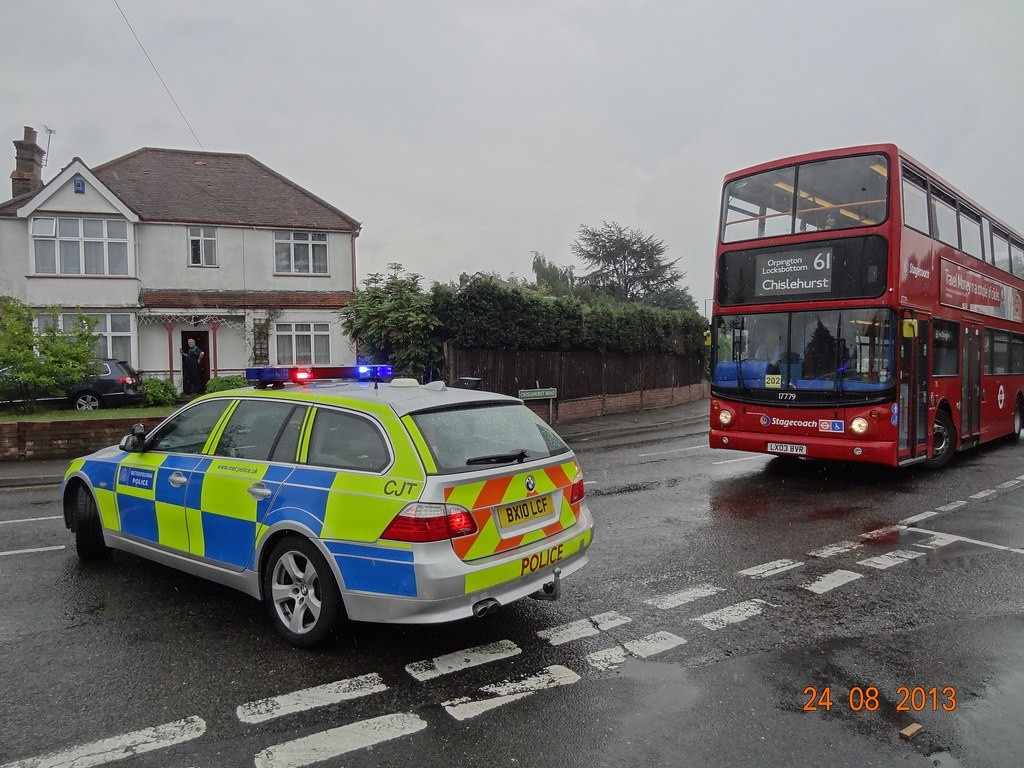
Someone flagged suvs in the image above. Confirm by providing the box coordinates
[0,355,150,411]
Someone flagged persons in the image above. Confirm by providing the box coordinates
[179,339,204,393]
[754,330,788,365]
[824,208,853,229]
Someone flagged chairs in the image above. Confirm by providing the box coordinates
[335,422,368,448]
[248,416,294,446]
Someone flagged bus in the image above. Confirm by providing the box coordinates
[703,142,1023,472]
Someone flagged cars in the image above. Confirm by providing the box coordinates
[59,365,597,650]
